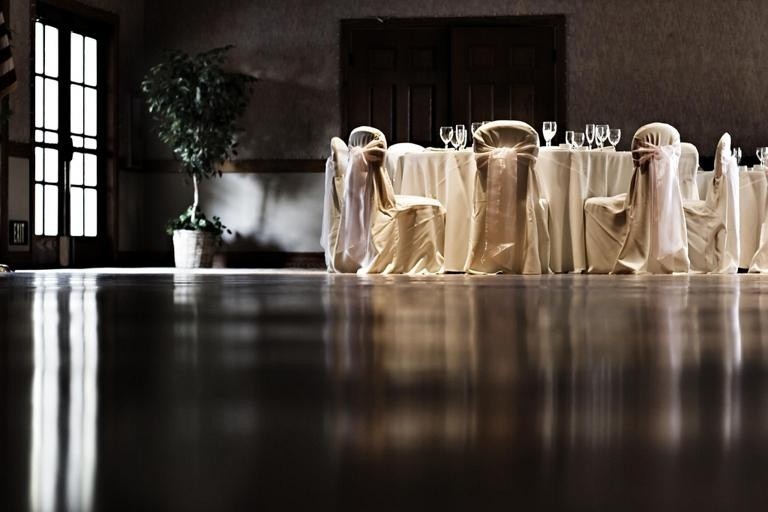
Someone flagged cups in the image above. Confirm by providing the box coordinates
[757,147,768,167]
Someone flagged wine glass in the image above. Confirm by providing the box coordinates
[542,121,621,152]
[439,121,489,151]
[732,147,742,167]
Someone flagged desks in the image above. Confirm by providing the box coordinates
[696,168,767,275]
[396,145,635,277]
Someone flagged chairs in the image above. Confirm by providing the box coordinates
[466,120,551,277]
[678,141,703,202]
[582,119,690,277]
[321,124,449,276]
[681,130,741,276]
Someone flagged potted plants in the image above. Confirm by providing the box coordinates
[141,42,255,269]
